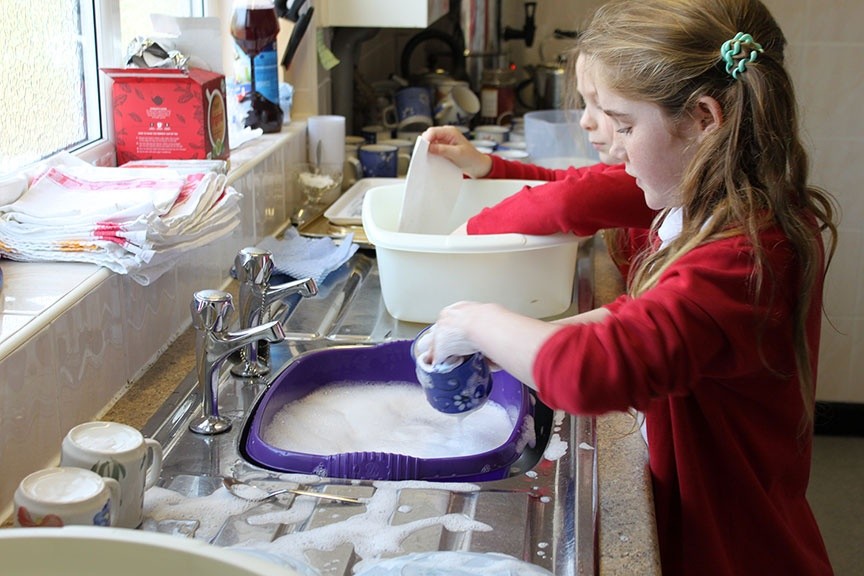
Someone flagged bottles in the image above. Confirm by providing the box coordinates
[253,40,280,106]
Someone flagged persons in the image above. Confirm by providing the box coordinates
[419,0,848,576]
[421,34,669,292]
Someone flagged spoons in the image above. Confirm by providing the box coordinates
[222,476,367,505]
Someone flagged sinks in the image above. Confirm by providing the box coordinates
[139,333,598,492]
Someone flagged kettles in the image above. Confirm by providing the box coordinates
[522,29,584,109]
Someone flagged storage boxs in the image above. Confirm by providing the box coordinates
[100,13,230,160]
[362,177,590,323]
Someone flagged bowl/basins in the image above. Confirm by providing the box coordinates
[523,110,601,171]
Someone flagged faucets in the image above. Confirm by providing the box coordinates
[187,289,285,435]
[230,247,317,379]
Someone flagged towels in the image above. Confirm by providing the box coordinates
[0,150,244,287]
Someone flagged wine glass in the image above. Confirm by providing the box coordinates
[230,1,279,94]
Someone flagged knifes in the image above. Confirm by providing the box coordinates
[272,0,314,69]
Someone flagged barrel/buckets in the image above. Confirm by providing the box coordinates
[449,0,503,89]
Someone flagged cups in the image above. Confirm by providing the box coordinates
[345,72,531,188]
[410,324,492,415]
[307,114,344,176]
[14,466,122,528]
[60,421,163,529]
